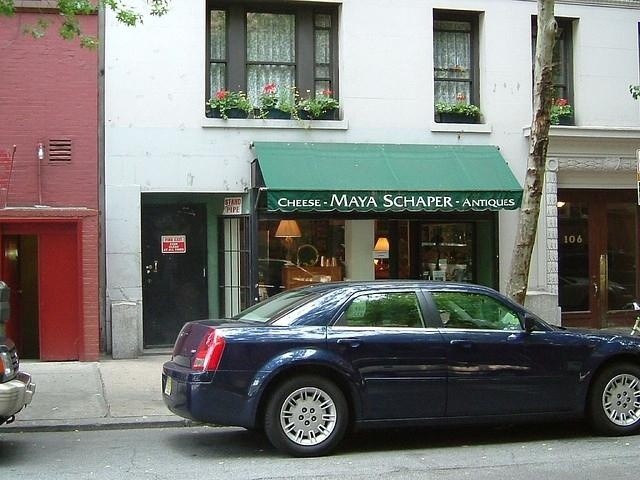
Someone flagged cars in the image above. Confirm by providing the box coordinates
[0,336,39,428]
[559,272,634,308]
[161,278,640,459]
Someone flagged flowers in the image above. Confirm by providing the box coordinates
[206,89,229,120]
[315,88,339,114]
[436,93,481,120]
[550,99,575,125]
[290,86,318,119]
[260,83,291,111]
[227,91,253,113]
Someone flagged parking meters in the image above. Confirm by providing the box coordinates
[0,281,11,337]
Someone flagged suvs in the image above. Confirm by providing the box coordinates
[258,258,322,296]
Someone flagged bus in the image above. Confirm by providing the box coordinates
[422,241,470,279]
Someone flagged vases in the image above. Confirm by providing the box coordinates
[297,108,312,120]
[559,117,574,126]
[211,107,221,120]
[263,108,291,119]
[440,114,480,123]
[321,108,340,121]
[226,110,249,118]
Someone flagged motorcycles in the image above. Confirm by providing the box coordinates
[630,301,640,337]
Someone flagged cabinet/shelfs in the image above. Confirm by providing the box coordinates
[283,266,343,289]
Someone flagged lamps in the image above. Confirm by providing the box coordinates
[374,238,389,270]
[274,220,302,244]
